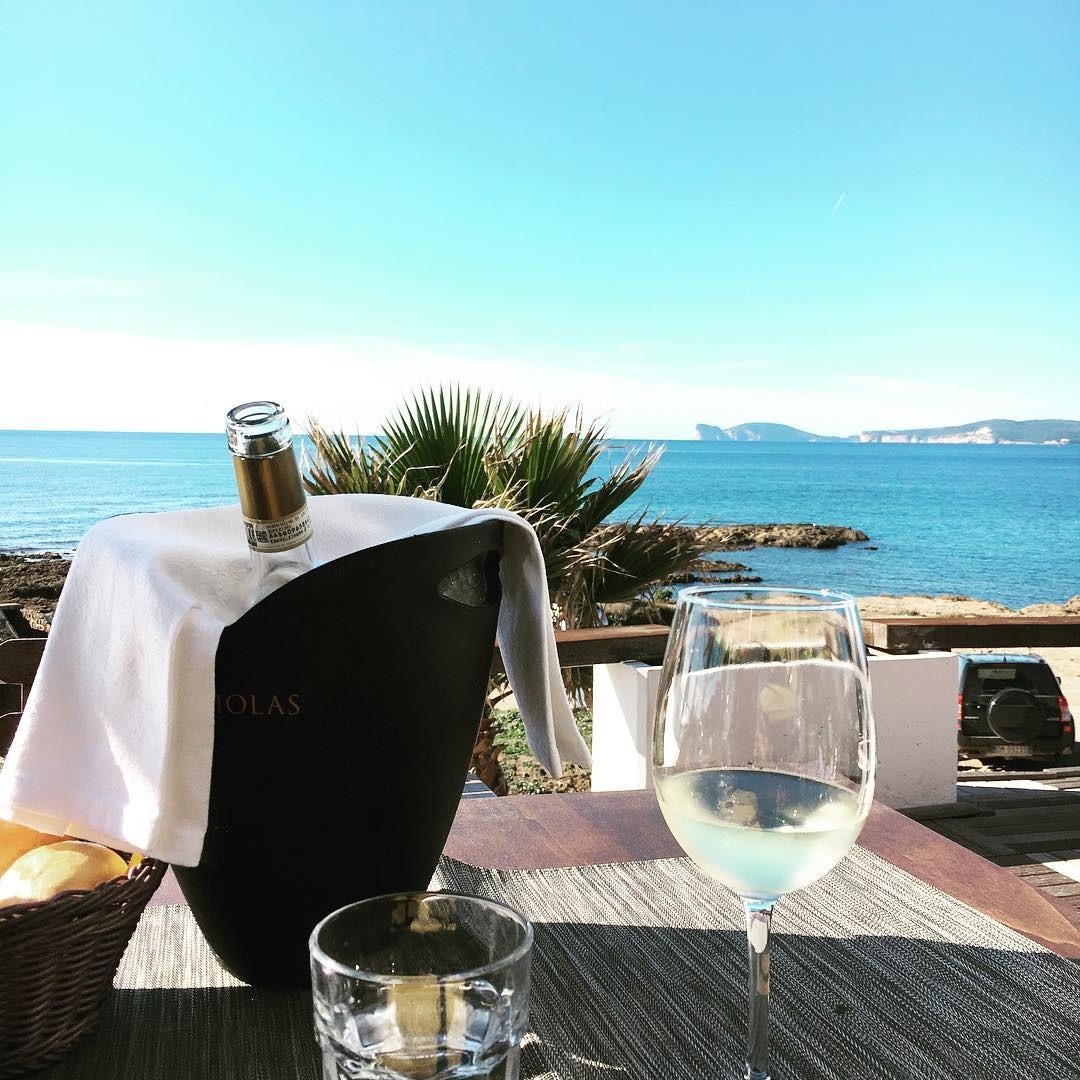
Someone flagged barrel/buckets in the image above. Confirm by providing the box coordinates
[167,520,502,985]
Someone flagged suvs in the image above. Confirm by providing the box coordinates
[956,652,1076,765]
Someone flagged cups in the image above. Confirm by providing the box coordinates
[307,896,537,1080]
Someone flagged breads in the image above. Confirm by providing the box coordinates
[1,818,132,907]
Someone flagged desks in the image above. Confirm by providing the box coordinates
[26,787,1079,1080]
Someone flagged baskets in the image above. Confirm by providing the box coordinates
[0,752,172,1078]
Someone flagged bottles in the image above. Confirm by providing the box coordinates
[224,401,323,611]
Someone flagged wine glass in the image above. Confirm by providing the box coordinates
[651,584,878,1079]
